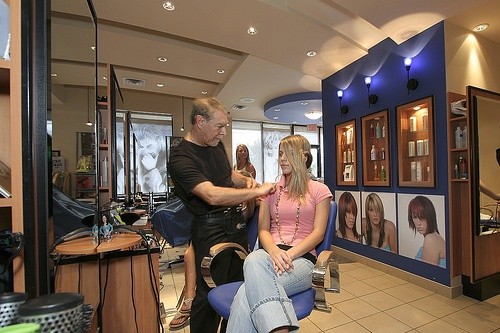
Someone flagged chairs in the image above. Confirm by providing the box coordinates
[201,198,339,333]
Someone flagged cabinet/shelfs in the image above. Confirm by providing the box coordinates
[97,94,111,193]
[360,108,389,187]
[449,117,466,182]
[395,92,436,189]
[335,119,356,188]
[0,1,27,301]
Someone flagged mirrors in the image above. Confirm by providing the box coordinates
[107,63,139,202]
[44,0,99,246]
[466,85,500,237]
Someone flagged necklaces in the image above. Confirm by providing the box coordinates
[274,186,301,245]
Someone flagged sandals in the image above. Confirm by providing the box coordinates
[169,297,194,327]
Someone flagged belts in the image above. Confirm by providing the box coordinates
[196,207,241,219]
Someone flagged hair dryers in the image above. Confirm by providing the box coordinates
[0,230,23,267]
[120,212,142,228]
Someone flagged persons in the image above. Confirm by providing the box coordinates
[233,145,256,188]
[116,124,167,195]
[226,135,333,333]
[407,196,446,269]
[361,193,397,255]
[168,97,276,333]
[142,171,256,328]
[263,132,281,183]
[335,192,360,243]
[169,171,255,328]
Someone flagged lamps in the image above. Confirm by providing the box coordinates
[364,77,379,111]
[336,90,349,120]
[404,58,418,96]
